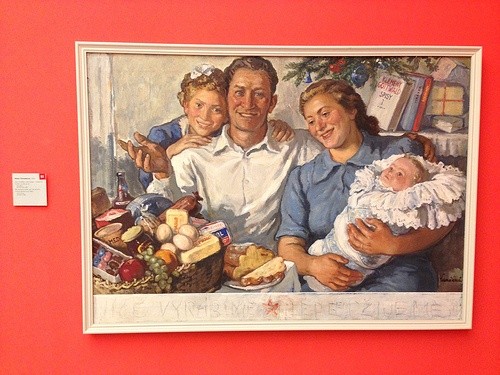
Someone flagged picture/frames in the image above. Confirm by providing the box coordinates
[74,41,482,335]
